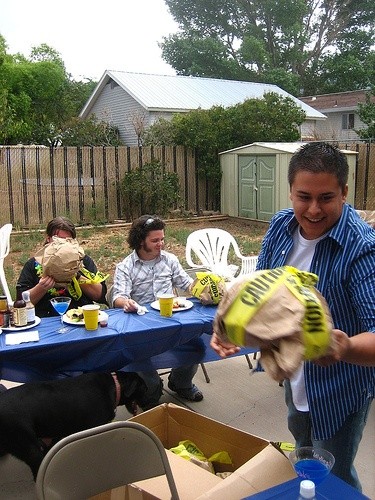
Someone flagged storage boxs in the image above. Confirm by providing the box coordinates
[83,402,298,500]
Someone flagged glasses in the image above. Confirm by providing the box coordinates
[142,218,158,228]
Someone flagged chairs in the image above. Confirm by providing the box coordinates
[184,229,259,293]
[37,421,179,500]
[0,224,15,309]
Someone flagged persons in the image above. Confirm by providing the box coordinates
[108,213,205,402]
[208,140,375,493]
[16,216,107,319]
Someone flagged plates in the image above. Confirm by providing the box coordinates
[62,310,109,325]
[150,299,194,311]
[0,316,41,331]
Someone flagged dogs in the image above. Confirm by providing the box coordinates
[0,368,165,486]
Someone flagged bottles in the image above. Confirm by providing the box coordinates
[23,291,35,325]
[0,296,9,328]
[10,305,15,326]
[13,284,27,327]
[297,479,316,500]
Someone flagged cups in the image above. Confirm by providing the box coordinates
[99,315,108,327]
[289,446,336,488]
[177,297,186,307]
[82,304,101,331]
[157,294,174,318]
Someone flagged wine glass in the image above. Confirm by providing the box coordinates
[50,297,72,334]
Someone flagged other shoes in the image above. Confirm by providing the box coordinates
[168,381,203,401]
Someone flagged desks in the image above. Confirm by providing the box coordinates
[240,468,372,500]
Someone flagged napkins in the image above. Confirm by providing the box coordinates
[123,298,149,315]
[5,330,40,346]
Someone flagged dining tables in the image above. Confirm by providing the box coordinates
[0,298,261,430]
